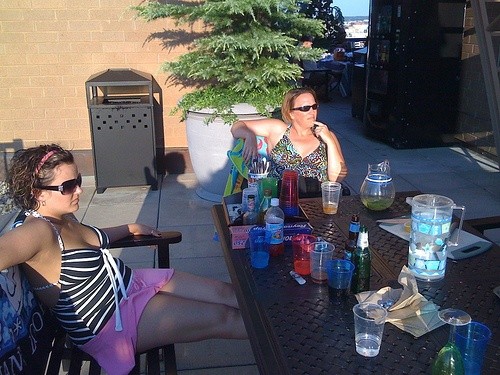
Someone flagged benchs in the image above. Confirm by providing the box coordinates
[0,207,182,375]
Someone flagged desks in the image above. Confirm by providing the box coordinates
[212,191,500,375]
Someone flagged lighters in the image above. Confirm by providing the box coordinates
[291,270,306,284]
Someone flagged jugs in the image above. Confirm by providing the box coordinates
[359,159,395,210]
[405,193,465,282]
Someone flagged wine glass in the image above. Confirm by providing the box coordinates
[428,308,472,375]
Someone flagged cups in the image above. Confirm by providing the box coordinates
[324,259,355,289]
[248,229,272,268]
[279,171,299,216]
[242,188,261,212]
[261,178,278,197]
[309,242,335,284]
[291,234,318,276]
[455,322,492,375]
[248,172,267,188]
[352,302,387,357]
[321,181,341,214]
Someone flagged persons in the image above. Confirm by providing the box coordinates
[0,144,249,375]
[295,34,349,103]
[231,87,347,198]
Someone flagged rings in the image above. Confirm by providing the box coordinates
[151,230,154,234]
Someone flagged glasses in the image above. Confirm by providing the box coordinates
[39,173,82,195]
[290,103,319,111]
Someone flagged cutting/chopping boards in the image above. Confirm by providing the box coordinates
[379,215,493,261]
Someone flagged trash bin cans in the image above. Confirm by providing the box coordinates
[84,66,166,195]
[350,46,369,122]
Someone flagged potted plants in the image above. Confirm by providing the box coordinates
[125,0,334,205]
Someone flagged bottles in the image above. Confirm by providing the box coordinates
[259,189,272,225]
[243,195,260,225]
[352,225,371,293]
[344,214,361,272]
[264,198,285,257]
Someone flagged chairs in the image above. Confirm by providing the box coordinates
[301,70,329,101]
[237,137,351,196]
